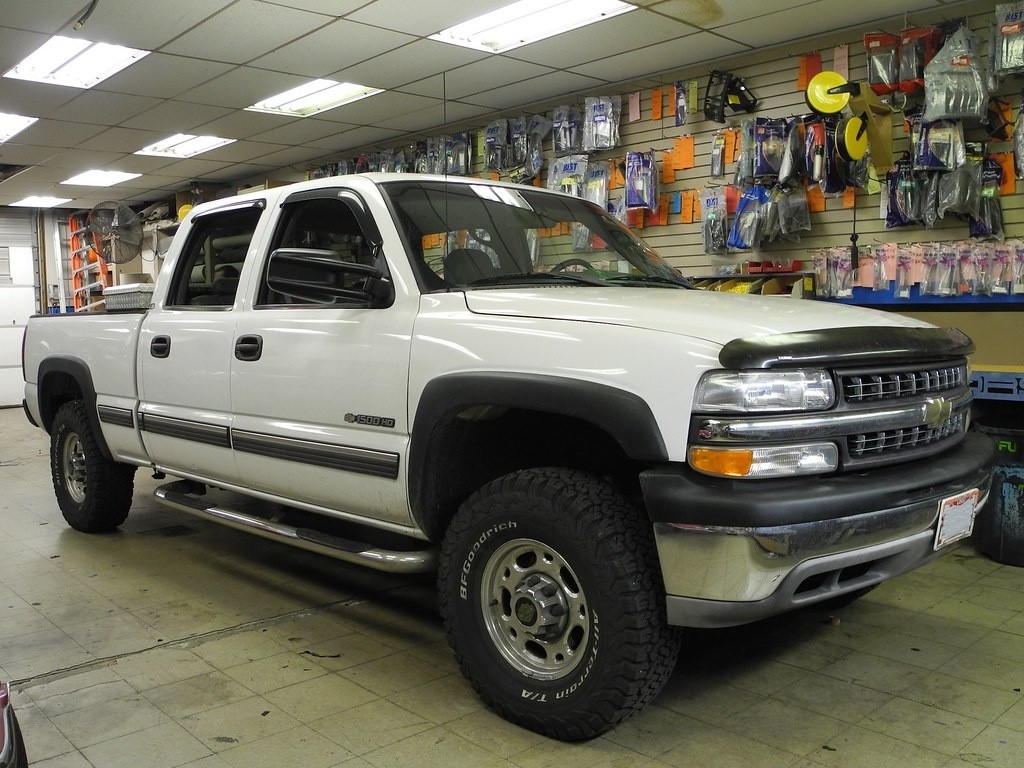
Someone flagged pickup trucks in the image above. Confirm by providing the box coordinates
[16,172,1000,746]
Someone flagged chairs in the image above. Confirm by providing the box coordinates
[443,249,497,284]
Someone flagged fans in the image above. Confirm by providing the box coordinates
[85,200,144,264]
